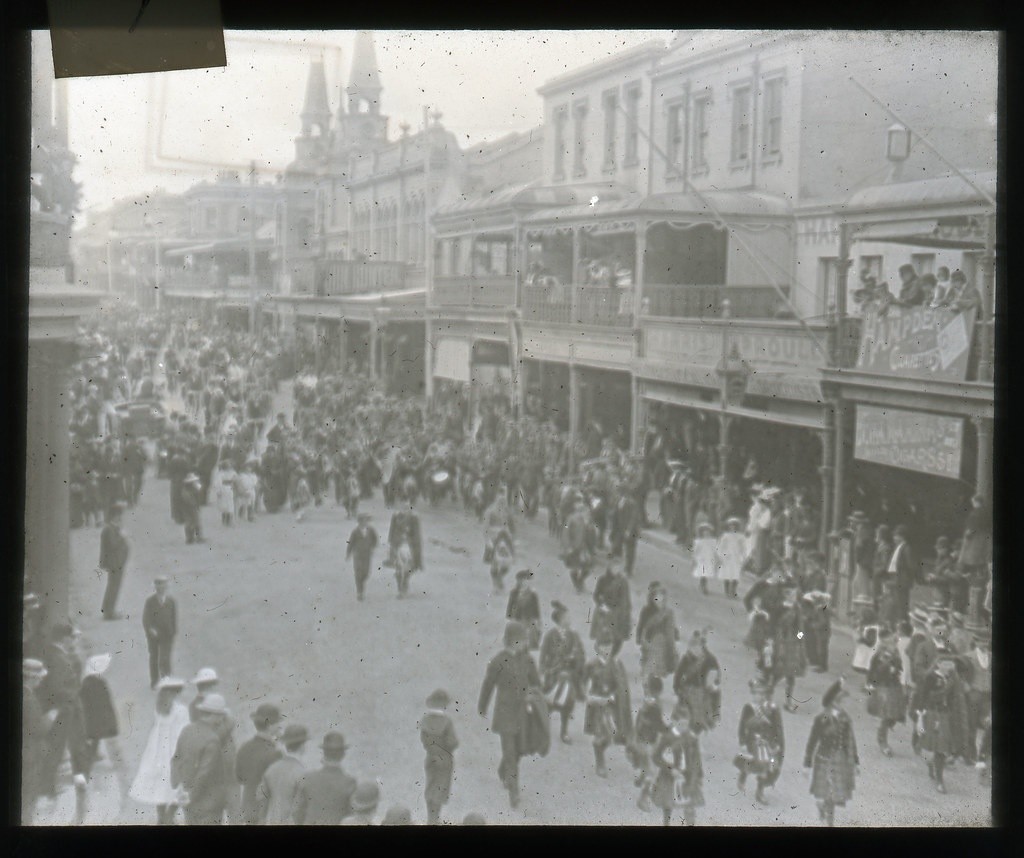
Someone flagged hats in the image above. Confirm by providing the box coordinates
[68,437,991,736]
[190,668,220,684]
[153,577,167,588]
[319,733,351,751]
[349,783,379,811]
[427,689,449,703]
[250,702,284,723]
[280,725,312,744]
[194,694,229,714]
[382,805,411,824]
[22,659,48,677]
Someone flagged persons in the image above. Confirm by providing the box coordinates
[22,310,993,825]
[854,263,984,383]
[523,257,632,287]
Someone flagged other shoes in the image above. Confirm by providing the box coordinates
[928,761,934,778]
[883,746,893,756]
[936,782,945,792]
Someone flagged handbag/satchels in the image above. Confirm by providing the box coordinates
[653,779,689,807]
